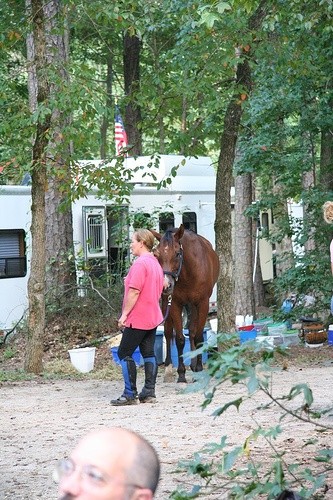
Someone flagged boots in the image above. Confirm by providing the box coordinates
[110,356,158,406]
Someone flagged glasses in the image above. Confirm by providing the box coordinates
[52,454,150,489]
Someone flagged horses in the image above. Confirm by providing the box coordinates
[146,223,220,392]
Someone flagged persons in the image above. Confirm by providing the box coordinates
[54,427,160,500]
[109,229,165,406]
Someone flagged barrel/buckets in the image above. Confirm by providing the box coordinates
[326,329,333,345]
[110,345,141,368]
[238,327,257,346]
[67,346,96,373]
[170,327,208,369]
[153,331,164,365]
